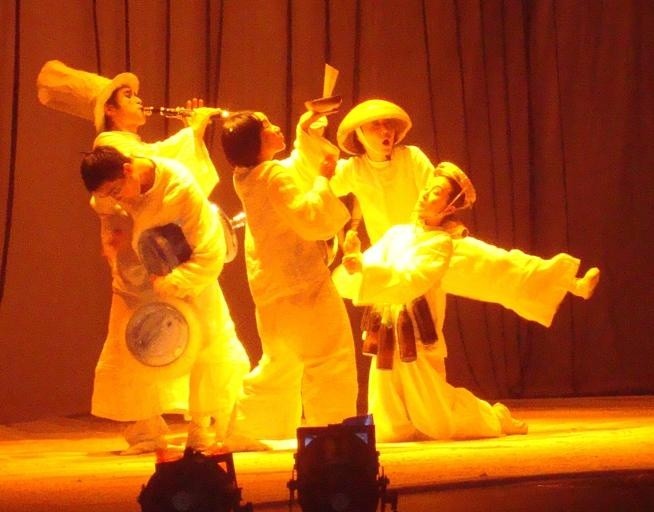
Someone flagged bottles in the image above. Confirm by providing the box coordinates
[357,294,439,372]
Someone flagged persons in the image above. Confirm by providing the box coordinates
[218,108,360,454]
[327,100,601,329]
[332,160,528,442]
[77,144,249,455]
[89,83,225,202]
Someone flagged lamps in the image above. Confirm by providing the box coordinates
[293,422,383,511]
[138,453,242,512]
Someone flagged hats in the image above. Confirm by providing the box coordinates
[434,162,477,208]
[117,298,202,385]
[337,100,411,156]
[37,61,140,133]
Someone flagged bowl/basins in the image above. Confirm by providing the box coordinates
[303,95,343,114]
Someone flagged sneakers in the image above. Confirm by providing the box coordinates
[571,268,599,300]
[119,437,269,455]
[495,402,528,434]
